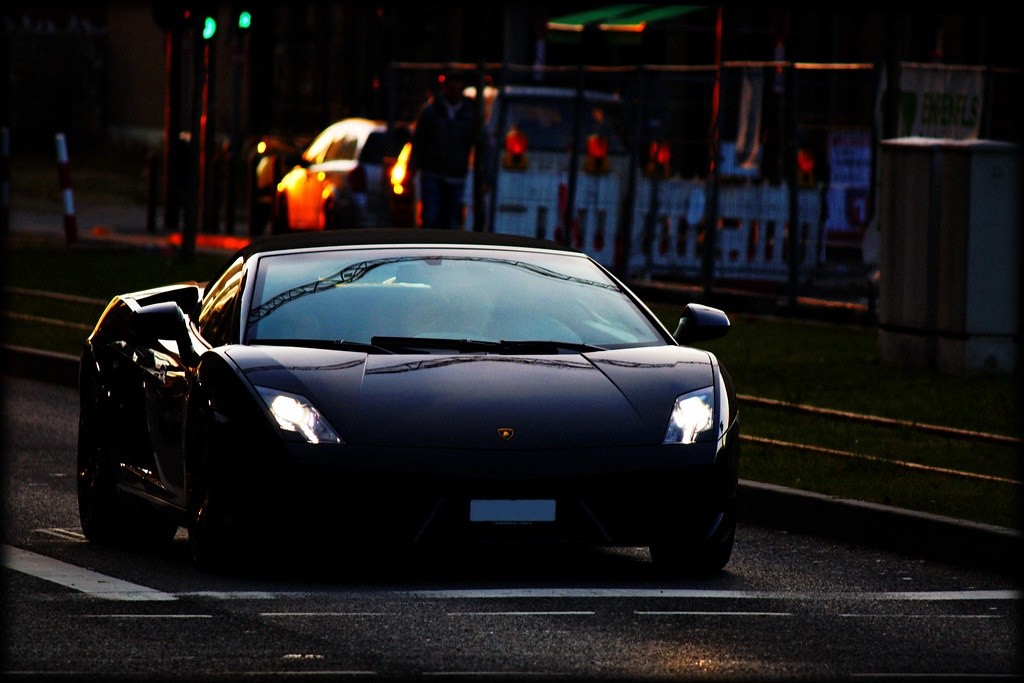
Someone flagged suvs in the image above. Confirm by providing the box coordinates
[271,115,471,238]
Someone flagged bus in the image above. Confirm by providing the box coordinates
[371,61,987,294]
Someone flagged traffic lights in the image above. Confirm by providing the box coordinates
[154,0,206,38]
[231,1,254,32]
[199,17,220,43]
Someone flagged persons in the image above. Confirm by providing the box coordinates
[409,73,500,227]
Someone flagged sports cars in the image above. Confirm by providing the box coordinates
[77,243,736,579]
